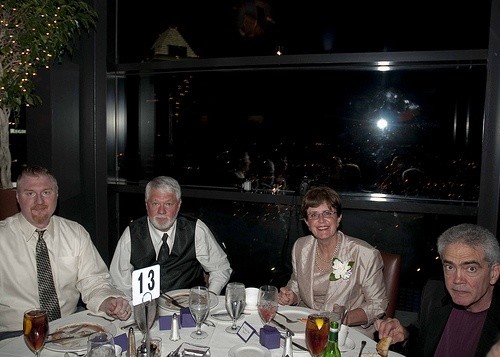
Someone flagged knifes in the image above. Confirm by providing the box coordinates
[280,336,309,351]
[271,319,294,336]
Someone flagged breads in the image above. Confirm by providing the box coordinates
[376,337,393,357]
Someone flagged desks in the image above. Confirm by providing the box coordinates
[0,295,408,357]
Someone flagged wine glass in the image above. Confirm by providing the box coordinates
[189,282,279,339]
[23,308,49,357]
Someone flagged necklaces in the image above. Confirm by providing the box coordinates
[315,233,341,272]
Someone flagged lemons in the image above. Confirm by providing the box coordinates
[23,314,32,334]
[316,319,324,330]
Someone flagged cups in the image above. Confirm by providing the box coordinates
[244,287,265,305]
[151,337,163,357]
[305,314,330,357]
[320,311,341,332]
[337,324,349,346]
[103,344,122,357]
[87,332,115,357]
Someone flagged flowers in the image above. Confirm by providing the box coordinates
[328,257,355,282]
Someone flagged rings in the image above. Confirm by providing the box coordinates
[125,310,128,315]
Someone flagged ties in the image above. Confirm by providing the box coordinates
[36,229,62,321]
[157,233,170,263]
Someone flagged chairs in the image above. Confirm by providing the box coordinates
[381,250,400,320]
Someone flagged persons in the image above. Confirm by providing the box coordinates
[222,144,439,195]
[374,223,500,357]
[279,185,390,341]
[110,176,233,298]
[0,165,132,342]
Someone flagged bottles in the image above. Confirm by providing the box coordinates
[128,327,137,357]
[282,330,293,357]
[321,321,342,357]
[169,313,181,342]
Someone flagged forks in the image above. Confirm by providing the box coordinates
[275,312,299,323]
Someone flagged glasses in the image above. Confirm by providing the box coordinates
[307,210,338,220]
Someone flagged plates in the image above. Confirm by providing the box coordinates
[119,317,157,333]
[228,343,272,357]
[243,306,258,311]
[155,289,219,313]
[261,306,322,335]
[42,316,117,352]
[209,308,245,322]
[338,337,355,352]
